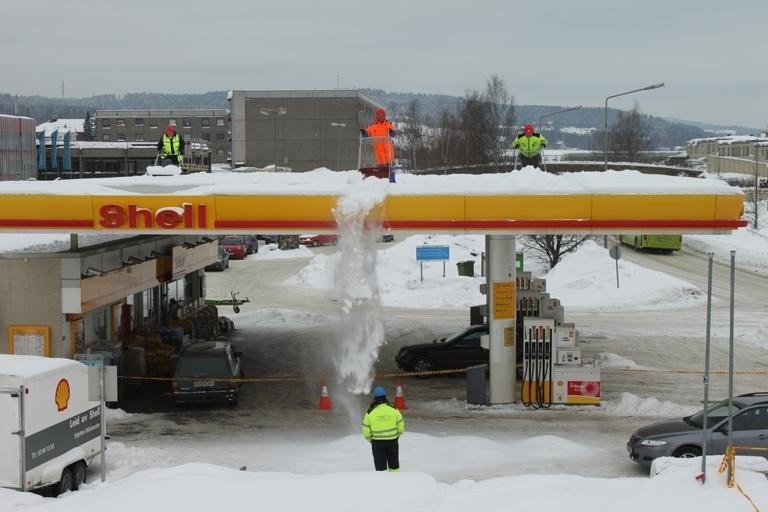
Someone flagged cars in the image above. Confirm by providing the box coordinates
[394,326,524,382]
[625,392,767,468]
[204,245,230,272]
[243,235,258,254]
[382,232,394,242]
[264,234,337,250]
[223,236,248,259]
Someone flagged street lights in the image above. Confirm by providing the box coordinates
[538,105,580,138]
[329,120,347,170]
[603,84,665,170]
[256,104,288,172]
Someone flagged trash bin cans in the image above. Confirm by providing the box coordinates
[456,261,475,277]
[516,251,523,272]
[466,364,488,405]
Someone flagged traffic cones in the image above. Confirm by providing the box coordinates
[318,380,333,411]
[392,380,407,411]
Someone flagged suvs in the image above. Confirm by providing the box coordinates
[170,335,243,408]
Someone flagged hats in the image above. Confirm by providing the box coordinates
[375,109,386,118]
[374,387,386,398]
[524,125,534,137]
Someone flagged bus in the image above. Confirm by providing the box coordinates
[609,233,682,255]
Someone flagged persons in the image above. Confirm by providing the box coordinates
[362,385,405,471]
[511,123,549,169]
[359,108,400,169]
[156,126,185,166]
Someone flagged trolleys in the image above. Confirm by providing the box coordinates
[356,126,396,185]
[512,144,546,174]
[148,153,184,177]
